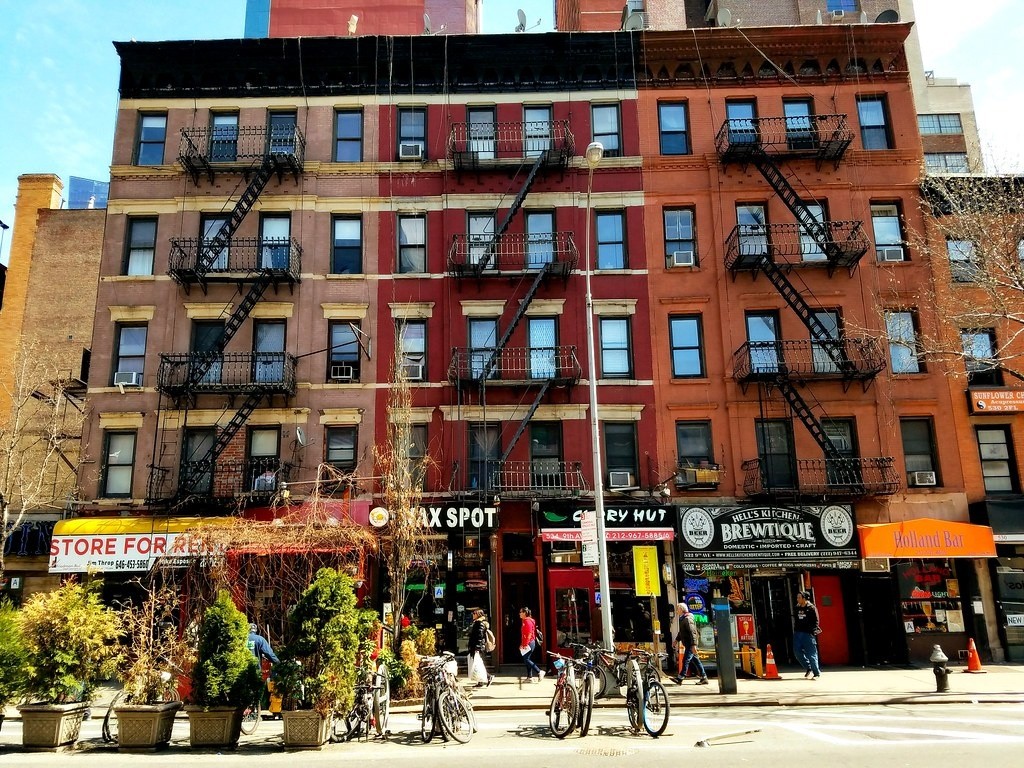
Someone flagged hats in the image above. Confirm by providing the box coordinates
[800,591,811,600]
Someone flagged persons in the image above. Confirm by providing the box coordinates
[519,607,546,683]
[669,603,709,685]
[793,591,822,680]
[245,623,280,713]
[630,603,652,642]
[468,609,495,688]
[184,608,202,632]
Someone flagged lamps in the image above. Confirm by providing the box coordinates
[492,494,502,507]
[592,567,600,584]
[660,489,671,505]
[488,533,500,553]
[532,502,540,511]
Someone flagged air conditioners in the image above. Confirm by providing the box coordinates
[914,471,936,485]
[400,364,422,380]
[399,143,421,160]
[608,471,631,486]
[860,558,891,573]
[883,247,903,261]
[113,371,139,387]
[331,366,354,378]
[831,9,845,21]
[672,250,694,269]
[255,475,277,492]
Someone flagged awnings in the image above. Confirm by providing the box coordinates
[227,541,349,555]
[48,516,235,572]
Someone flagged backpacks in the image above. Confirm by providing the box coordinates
[530,619,543,647]
[480,623,496,652]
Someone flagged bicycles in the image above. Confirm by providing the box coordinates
[626,649,670,736]
[567,641,616,737]
[329,664,389,744]
[99,654,262,745]
[579,640,661,700]
[546,649,587,738]
[417,650,477,744]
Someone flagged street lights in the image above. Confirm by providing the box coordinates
[585,141,622,699]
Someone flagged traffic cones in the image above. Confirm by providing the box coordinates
[963,638,988,674]
[677,645,691,677]
[762,643,783,680]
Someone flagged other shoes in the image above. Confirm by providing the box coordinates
[697,679,709,685]
[537,670,545,682]
[805,668,812,677]
[523,677,534,683]
[669,678,682,685]
[487,675,494,687]
[472,683,485,689]
[812,673,822,680]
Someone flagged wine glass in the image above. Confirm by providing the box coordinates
[743,622,749,635]
[921,601,935,628]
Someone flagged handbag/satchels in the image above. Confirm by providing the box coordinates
[467,651,487,683]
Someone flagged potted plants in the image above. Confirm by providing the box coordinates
[109,582,195,747]
[183,588,259,748]
[15,587,127,749]
[277,567,373,750]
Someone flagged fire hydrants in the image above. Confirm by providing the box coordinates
[929,644,954,693]
